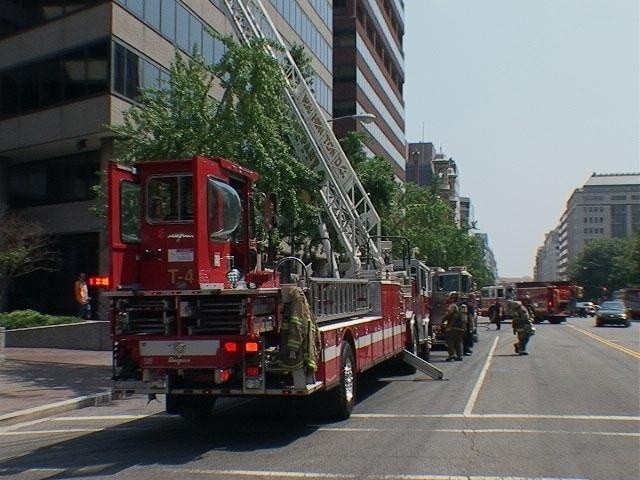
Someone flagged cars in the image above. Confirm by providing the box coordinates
[575,301,601,318]
[597,300,631,326]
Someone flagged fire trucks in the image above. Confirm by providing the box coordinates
[481,280,574,325]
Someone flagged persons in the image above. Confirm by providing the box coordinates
[74,273,92,320]
[495,298,506,330]
[441,290,478,361]
[512,295,537,355]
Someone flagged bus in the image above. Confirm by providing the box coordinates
[612,287,639,318]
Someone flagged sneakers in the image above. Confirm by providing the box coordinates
[446,357,463,362]
[514,344,527,355]
